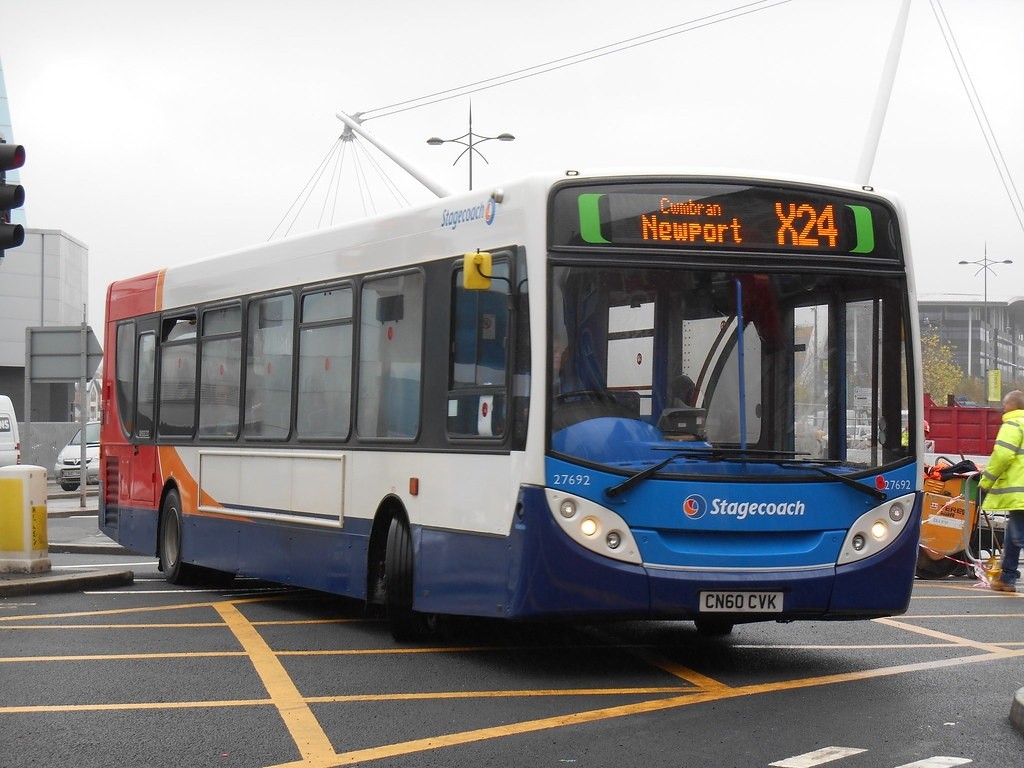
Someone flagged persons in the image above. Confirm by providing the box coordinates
[978,390,1024,593]
[900,421,930,446]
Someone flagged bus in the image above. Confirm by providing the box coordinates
[97,172,924,644]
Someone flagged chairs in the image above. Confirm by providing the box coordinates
[552,389,641,437]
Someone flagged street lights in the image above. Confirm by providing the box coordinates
[425,97,515,192]
[958,243,1013,405]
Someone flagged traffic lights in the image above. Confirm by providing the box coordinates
[0,137,25,257]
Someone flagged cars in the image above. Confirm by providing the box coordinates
[53,420,101,492]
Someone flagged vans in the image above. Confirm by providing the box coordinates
[0,394,22,467]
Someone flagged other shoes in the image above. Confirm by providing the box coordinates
[990,579,1016,592]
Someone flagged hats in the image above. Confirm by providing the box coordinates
[924,420,930,432]
